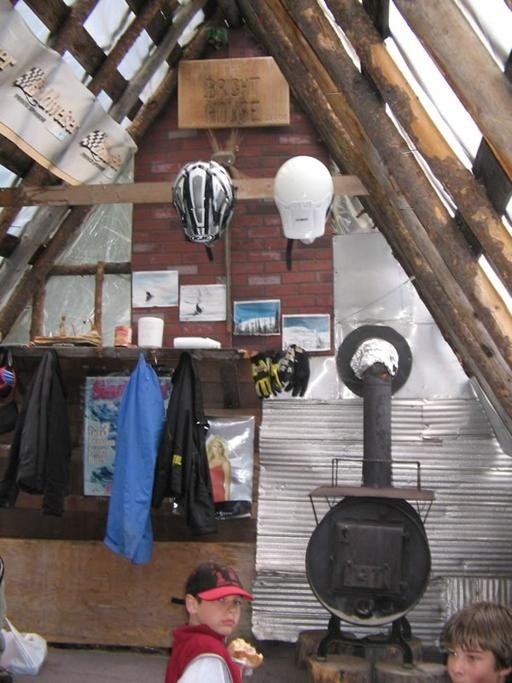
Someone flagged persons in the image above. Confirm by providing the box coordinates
[165,562,255,683]
[440,601,512,683]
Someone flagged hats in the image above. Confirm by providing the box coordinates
[184,560,255,604]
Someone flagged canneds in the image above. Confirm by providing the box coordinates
[114,326,130,348]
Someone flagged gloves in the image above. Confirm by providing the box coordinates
[248,342,311,399]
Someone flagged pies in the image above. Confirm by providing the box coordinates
[226,637,263,670]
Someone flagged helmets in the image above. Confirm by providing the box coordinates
[168,156,238,246]
[271,152,335,240]
